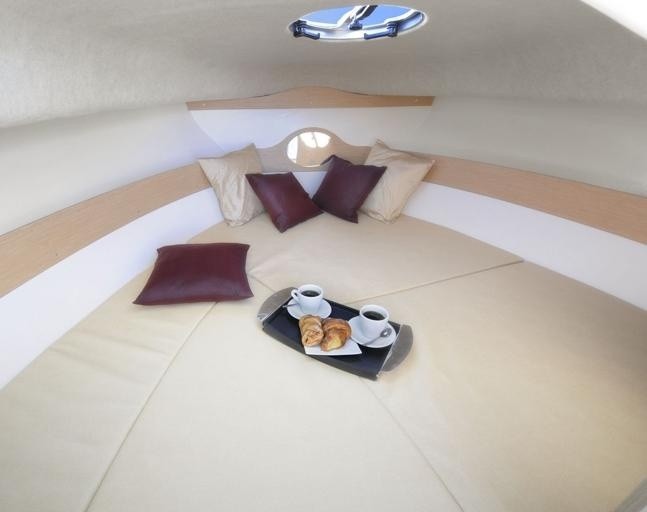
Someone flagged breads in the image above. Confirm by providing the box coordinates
[299,314,324,346]
[320,317,352,351]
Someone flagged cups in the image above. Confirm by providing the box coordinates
[291,285,323,315]
[359,306,389,339]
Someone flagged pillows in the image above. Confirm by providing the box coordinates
[197,138,437,235]
[132,243,252,309]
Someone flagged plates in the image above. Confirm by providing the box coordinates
[288,298,332,320]
[348,316,397,347]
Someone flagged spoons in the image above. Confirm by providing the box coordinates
[362,329,392,347]
